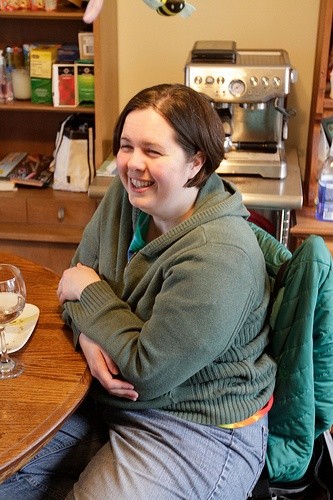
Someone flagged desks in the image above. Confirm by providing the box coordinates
[88,146,304,247]
[0,249,92,482]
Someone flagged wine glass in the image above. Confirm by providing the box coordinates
[0,264,27,380]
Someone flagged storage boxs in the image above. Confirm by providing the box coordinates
[29,33,96,108]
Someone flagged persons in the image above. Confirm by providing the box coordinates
[0,83,277,500]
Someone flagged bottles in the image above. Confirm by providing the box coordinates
[316,141,332,222]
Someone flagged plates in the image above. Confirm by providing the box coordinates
[0,304,40,354]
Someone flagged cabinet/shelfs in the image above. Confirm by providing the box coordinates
[290,0,333,257]
[0,0,119,275]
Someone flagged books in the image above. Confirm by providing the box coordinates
[0,151,54,187]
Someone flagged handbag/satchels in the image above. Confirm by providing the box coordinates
[49,113,95,193]
